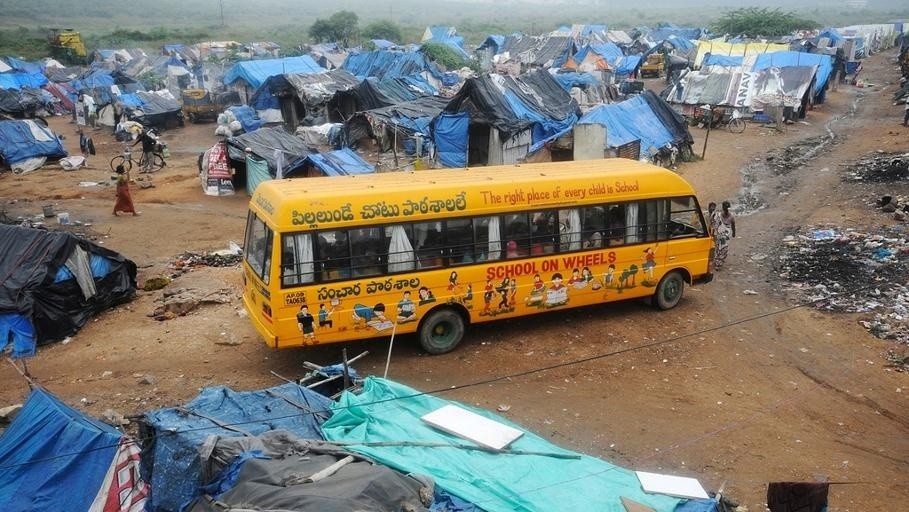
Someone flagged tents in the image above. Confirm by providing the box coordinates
[0,220,137,360]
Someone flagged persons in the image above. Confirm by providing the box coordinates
[702,200,735,271]
[112,165,141,216]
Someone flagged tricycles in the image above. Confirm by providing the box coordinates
[693,102,746,135]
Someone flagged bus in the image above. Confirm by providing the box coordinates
[238,156,720,358]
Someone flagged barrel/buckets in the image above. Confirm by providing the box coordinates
[44,206,54,217]
[124,153,131,160]
[57,213,68,223]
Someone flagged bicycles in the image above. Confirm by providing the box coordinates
[108,145,167,174]
